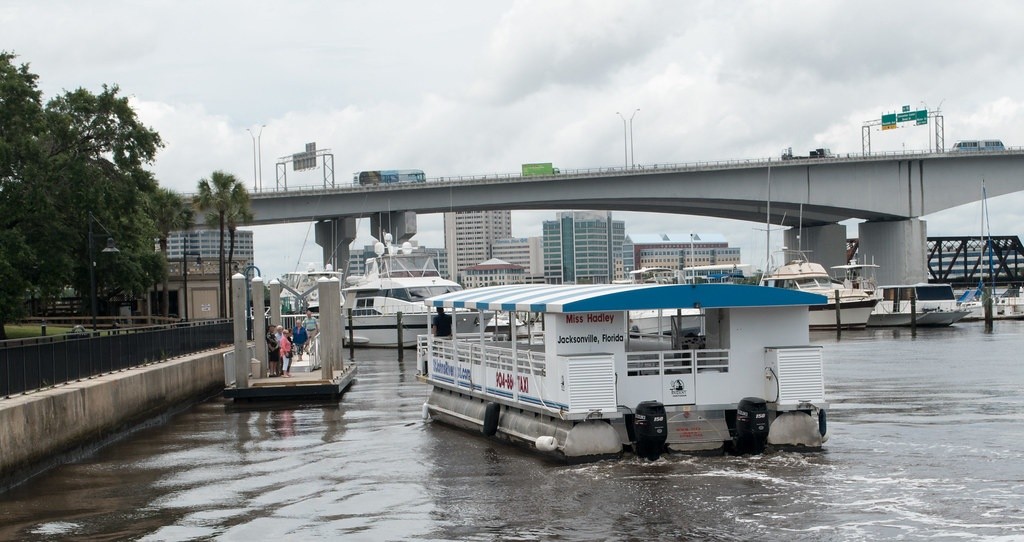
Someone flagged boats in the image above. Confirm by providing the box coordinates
[414,281,829,461]
[273,198,703,349]
[830,251,975,327]
[759,202,885,329]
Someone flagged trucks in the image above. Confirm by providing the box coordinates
[522,162,560,178]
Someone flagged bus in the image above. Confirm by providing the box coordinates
[352,169,426,189]
[948,138,1005,154]
[683,264,758,285]
[628,266,674,285]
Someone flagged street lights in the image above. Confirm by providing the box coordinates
[617,111,627,170]
[689,232,701,283]
[182,236,202,319]
[88,209,120,331]
[258,124,267,193]
[247,129,257,193]
[629,108,641,169]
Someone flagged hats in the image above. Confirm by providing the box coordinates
[268,324,276,328]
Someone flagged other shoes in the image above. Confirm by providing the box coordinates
[281,374,289,378]
[269,374,278,377]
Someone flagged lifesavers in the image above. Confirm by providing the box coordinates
[311,290,318,300]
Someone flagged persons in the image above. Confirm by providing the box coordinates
[72,321,85,333]
[302,311,318,355]
[112,323,120,335]
[272,325,296,378]
[266,324,279,377]
[432,307,452,338]
[292,320,308,362]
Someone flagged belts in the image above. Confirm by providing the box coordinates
[307,328,315,331]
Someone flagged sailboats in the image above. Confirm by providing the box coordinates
[952,178,1024,321]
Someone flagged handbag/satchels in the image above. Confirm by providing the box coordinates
[286,351,293,358]
